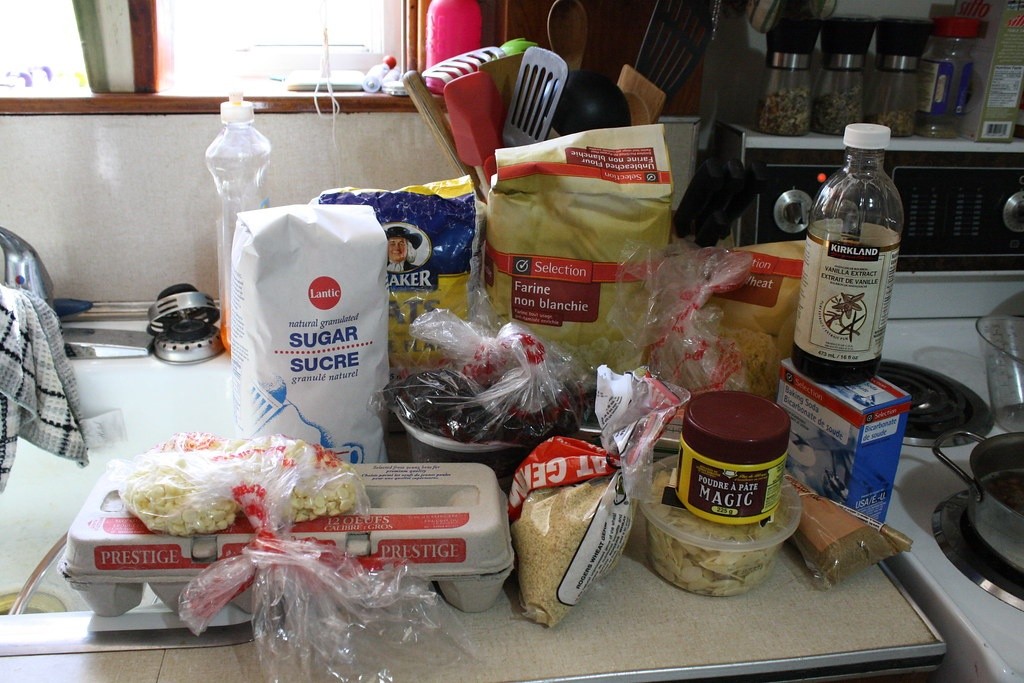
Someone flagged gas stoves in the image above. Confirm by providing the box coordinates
[703,117,1024,683]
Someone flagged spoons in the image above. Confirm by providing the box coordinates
[547,0,589,79]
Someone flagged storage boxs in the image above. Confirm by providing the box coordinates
[777,358,912,523]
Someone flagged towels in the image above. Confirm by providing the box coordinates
[1,285,90,493]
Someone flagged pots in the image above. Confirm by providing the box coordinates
[934,431,1024,573]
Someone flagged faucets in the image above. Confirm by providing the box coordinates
[0,227,56,302]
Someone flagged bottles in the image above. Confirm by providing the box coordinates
[205,86,284,358]
[426,0,483,95]
[361,56,397,92]
[790,123,904,387]
[751,10,979,142]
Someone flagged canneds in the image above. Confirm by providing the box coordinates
[675,390,791,525]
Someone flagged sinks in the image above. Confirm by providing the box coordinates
[0,315,287,658]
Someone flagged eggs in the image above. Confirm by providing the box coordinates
[379,489,477,509]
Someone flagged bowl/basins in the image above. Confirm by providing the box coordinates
[973,314,1024,431]
